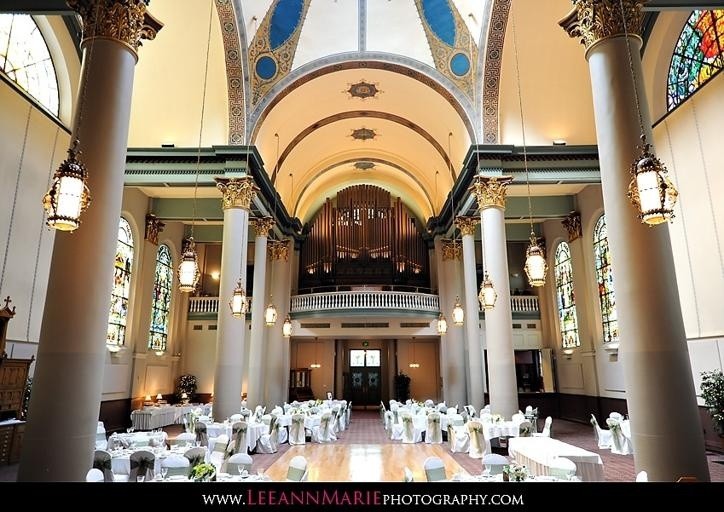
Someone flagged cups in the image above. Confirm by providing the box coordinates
[196,441,201,448]
[152,428,162,434]
[136,475,145,482]
[156,474,161,480]
[257,468,265,480]
[242,470,248,477]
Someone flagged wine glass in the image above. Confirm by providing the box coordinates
[237,464,245,477]
[161,468,168,480]
[126,427,135,435]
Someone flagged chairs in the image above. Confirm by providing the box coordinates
[85,398,353,483]
[379,398,630,483]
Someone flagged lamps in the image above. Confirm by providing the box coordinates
[42,0,93,235]
[430,12,501,335]
